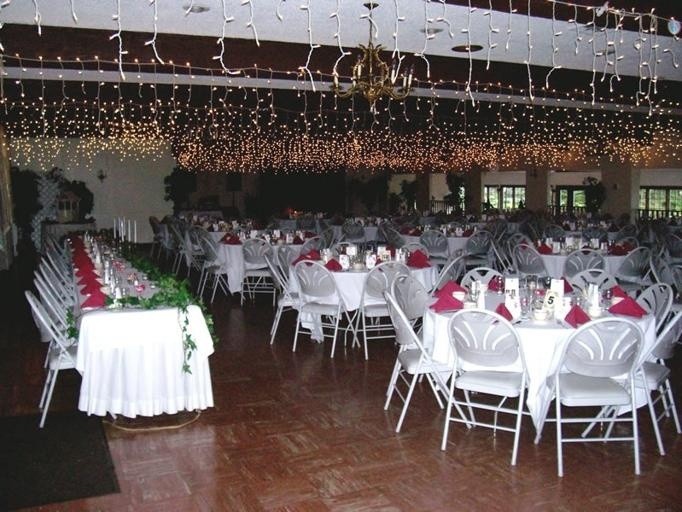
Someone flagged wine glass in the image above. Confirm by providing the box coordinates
[161,207,411,275]
[68,227,177,316]
[451,272,613,326]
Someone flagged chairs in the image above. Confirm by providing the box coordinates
[25,232,118,428]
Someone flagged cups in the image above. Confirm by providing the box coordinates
[413,211,682,258]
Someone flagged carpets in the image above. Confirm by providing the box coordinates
[0,409,120,512]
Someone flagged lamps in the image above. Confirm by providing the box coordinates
[329,3,414,110]
